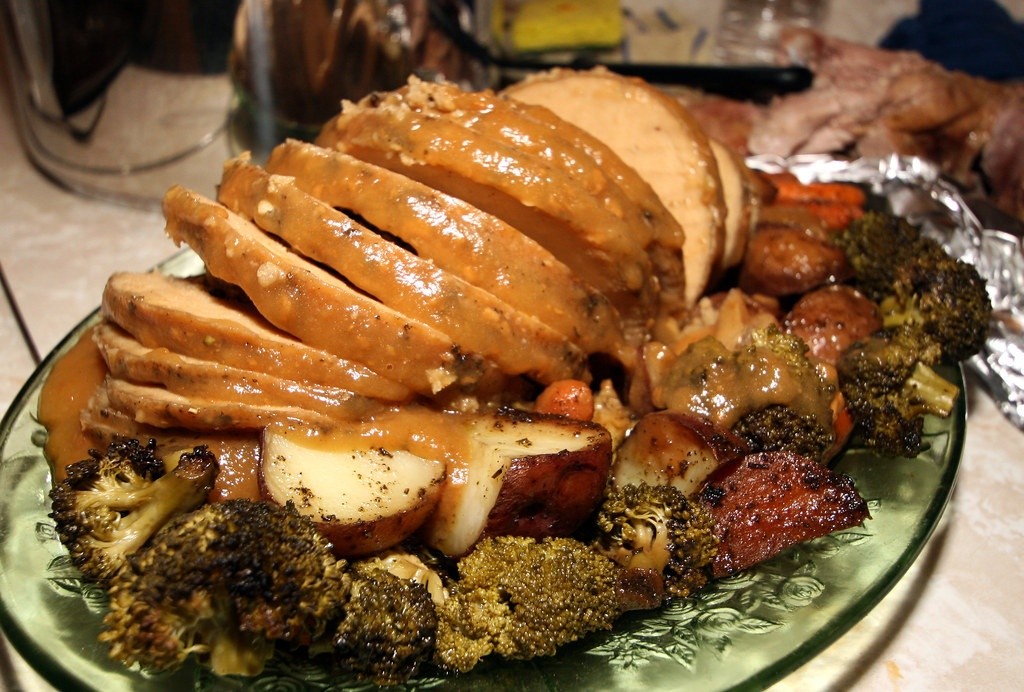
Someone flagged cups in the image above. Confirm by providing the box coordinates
[714,0,814,67]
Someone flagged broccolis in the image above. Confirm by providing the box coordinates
[52,210,992,686]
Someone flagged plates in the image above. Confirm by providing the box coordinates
[0,243,965,692]
